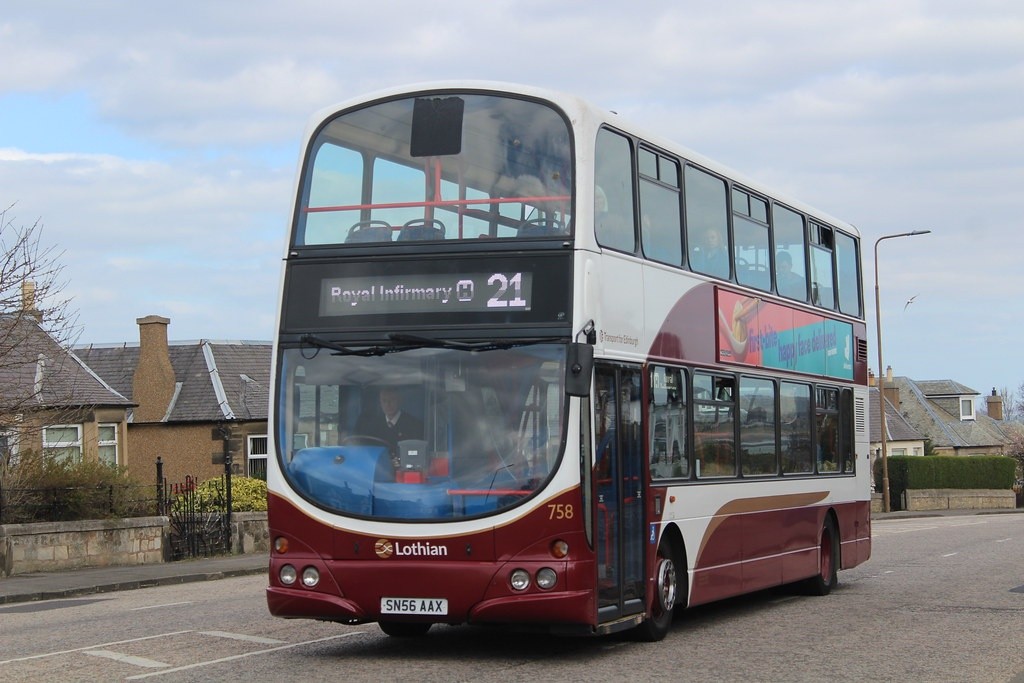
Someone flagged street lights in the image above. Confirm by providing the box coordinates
[872,230,933,513]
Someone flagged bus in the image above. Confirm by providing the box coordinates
[266,76,873,643]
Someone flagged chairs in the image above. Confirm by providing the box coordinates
[651,420,820,480]
[344,216,826,303]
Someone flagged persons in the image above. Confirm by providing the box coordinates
[341,386,425,444]
[567,184,611,248]
[701,224,730,279]
[776,251,804,301]
